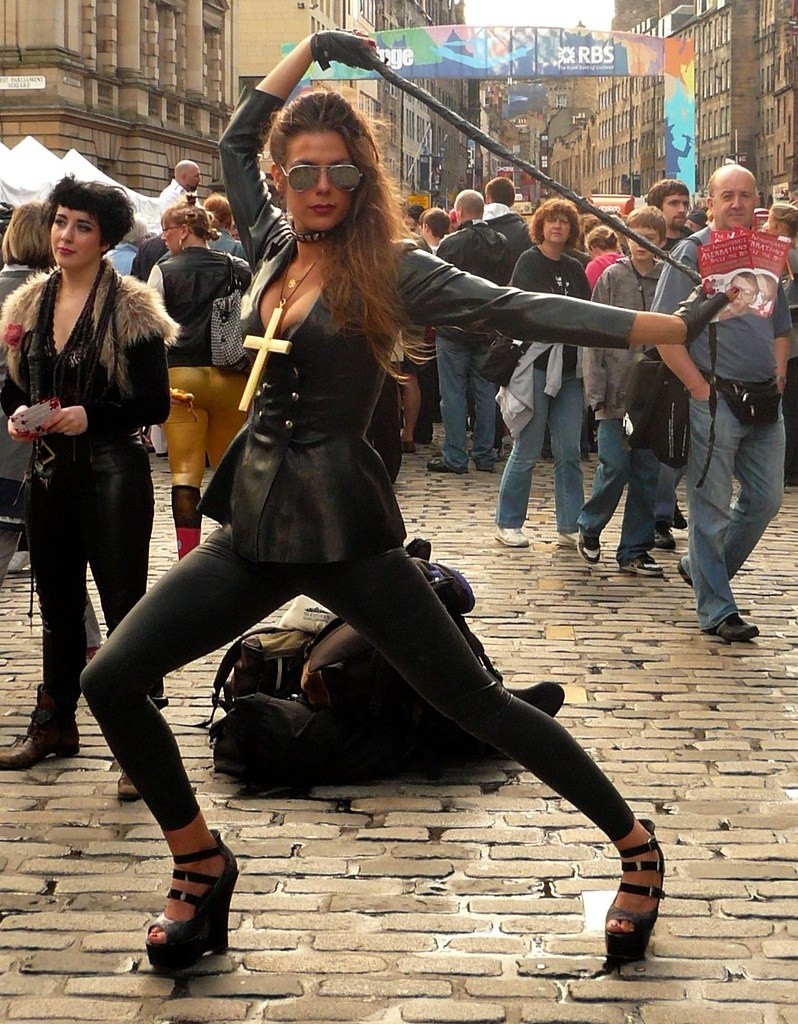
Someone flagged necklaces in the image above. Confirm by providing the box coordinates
[239,244,320,412]
[287,279,297,289]
[290,220,338,242]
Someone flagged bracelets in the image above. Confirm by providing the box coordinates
[778,375,788,384]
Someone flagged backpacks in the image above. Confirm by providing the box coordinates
[202,539,565,796]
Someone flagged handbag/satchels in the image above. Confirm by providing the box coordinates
[480,337,530,387]
[710,374,781,426]
[210,254,256,375]
[621,358,691,469]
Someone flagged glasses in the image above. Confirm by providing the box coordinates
[277,157,364,194]
[161,226,183,233]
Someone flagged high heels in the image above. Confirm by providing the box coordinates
[145,828,239,967]
[605,818,665,960]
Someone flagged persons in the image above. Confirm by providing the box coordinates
[756,274,777,308]
[0,172,180,803]
[0,160,798,665]
[718,271,762,322]
[79,30,740,971]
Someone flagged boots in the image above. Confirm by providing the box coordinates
[0,689,80,771]
[117,770,140,803]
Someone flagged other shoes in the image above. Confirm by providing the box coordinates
[426,458,468,473]
[399,437,416,453]
[474,461,494,471]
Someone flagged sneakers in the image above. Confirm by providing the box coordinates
[494,523,529,547]
[707,613,759,641]
[677,558,693,589]
[654,523,676,550]
[619,553,663,577]
[557,525,601,546]
[576,523,601,564]
[672,501,687,527]
